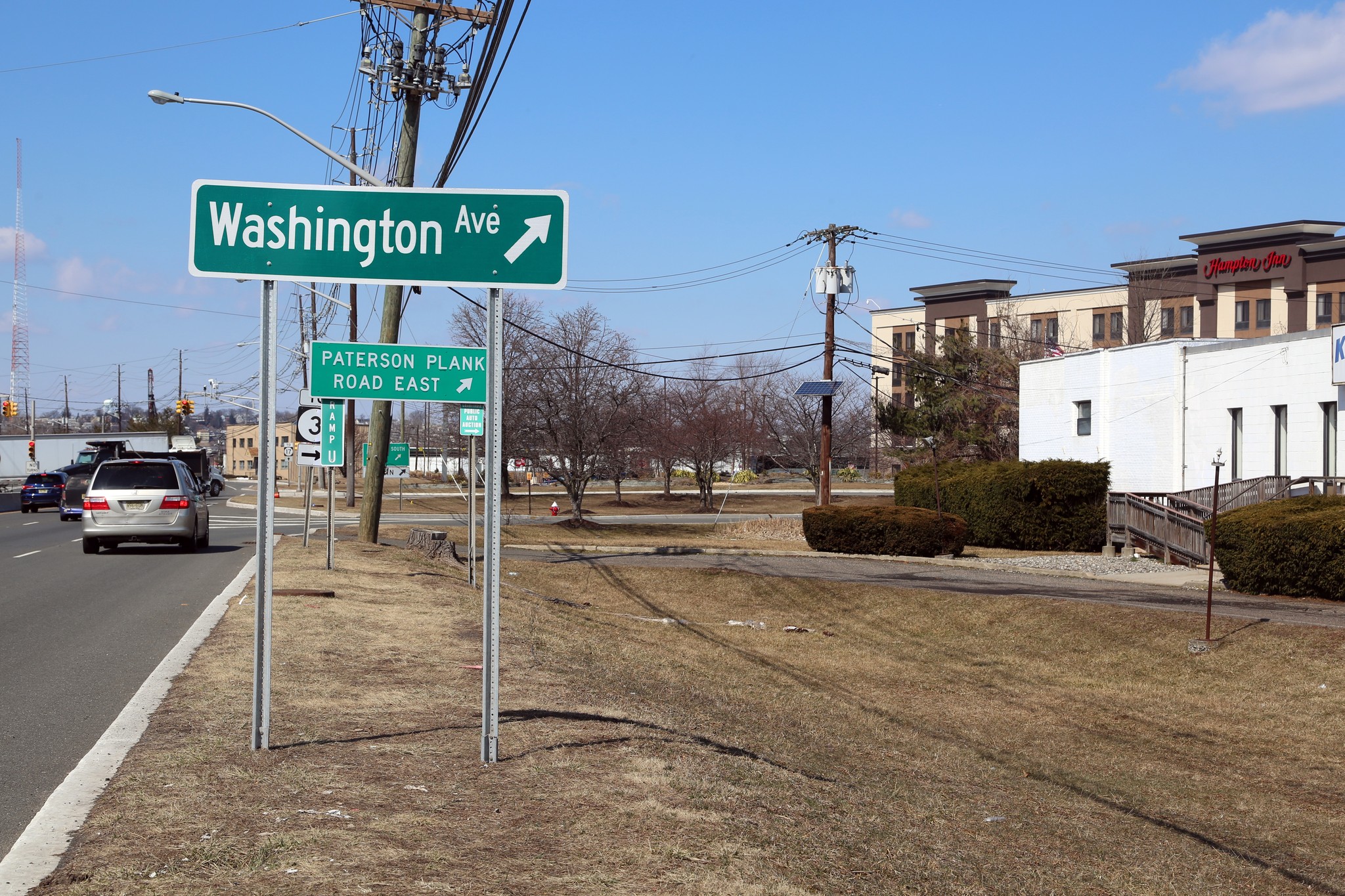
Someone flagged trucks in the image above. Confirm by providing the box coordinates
[200,471,226,497]
[206,458,224,476]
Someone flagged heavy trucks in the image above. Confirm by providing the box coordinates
[49,439,209,501]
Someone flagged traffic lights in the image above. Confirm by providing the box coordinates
[175,400,195,416]
[28,440,35,458]
[2,400,18,417]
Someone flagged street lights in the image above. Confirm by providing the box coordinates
[921,434,949,556]
[146,89,421,543]
[1205,447,1227,640]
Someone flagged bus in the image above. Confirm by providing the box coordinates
[743,454,870,479]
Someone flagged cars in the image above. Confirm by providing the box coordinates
[59,474,93,521]
[20,472,69,514]
[541,466,737,485]
